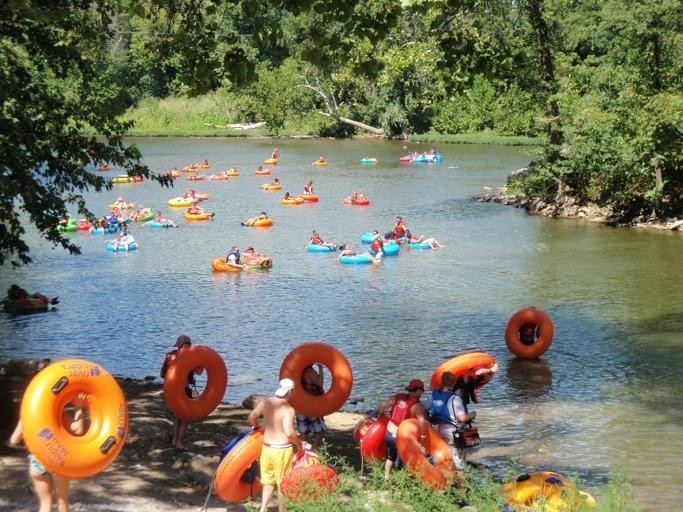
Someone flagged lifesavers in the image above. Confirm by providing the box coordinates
[263,158,279,165]
[20,359,128,478]
[165,164,239,181]
[281,194,318,205]
[354,413,389,462]
[212,252,273,273]
[505,308,553,358]
[360,159,376,164]
[266,185,281,190]
[344,198,369,206]
[312,161,329,166]
[254,218,272,225]
[215,427,263,501]
[169,193,209,220]
[256,168,271,176]
[279,343,353,416]
[500,472,581,512]
[308,231,435,264]
[400,154,443,163]
[55,203,174,253]
[431,352,496,391]
[163,346,227,421]
[281,465,338,502]
[2,297,48,315]
[111,174,142,184]
[397,419,458,490]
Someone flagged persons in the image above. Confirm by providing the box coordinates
[9,392,88,512]
[160,335,204,451]
[247,363,480,512]
[519,306,536,344]
[55,148,448,270]
[0,285,60,305]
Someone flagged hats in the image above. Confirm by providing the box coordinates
[274,377,294,397]
[404,379,424,392]
[174,335,191,348]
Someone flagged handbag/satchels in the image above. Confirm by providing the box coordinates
[452,426,480,449]
